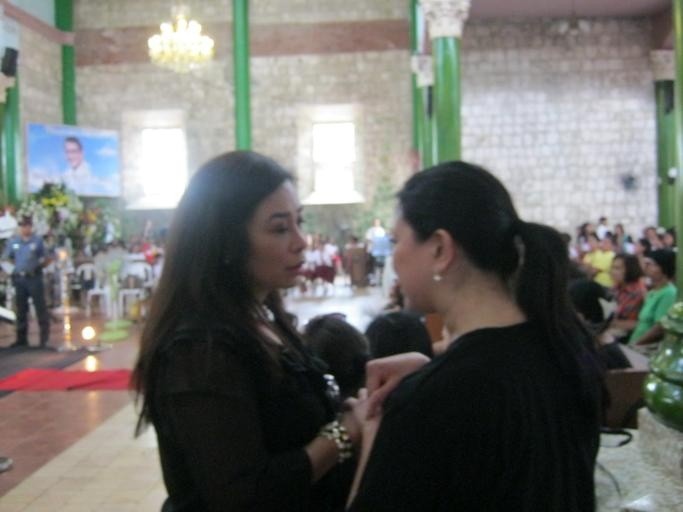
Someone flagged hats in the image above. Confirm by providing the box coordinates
[17,214,32,226]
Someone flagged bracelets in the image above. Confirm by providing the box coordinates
[319,420,356,466]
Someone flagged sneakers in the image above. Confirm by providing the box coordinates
[10,339,28,348]
[0,457,14,473]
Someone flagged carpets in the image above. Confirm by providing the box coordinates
[0,344,137,397]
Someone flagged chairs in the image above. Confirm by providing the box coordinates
[76,260,153,324]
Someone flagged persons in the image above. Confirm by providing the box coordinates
[560,212,683,432]
[2,215,55,352]
[55,134,94,199]
[341,160,614,511]
[300,310,369,400]
[130,148,364,512]
[281,216,390,301]
[361,311,437,367]
